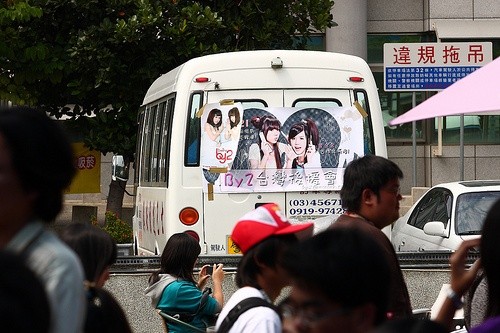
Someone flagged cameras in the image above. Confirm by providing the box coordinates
[206,266,219,275]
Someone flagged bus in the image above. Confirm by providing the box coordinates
[112,49,393,263]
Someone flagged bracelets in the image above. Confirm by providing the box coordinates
[444,287,463,309]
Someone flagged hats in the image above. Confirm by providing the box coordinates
[230,203,314,255]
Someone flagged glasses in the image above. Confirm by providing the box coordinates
[374,188,401,196]
[281,300,353,321]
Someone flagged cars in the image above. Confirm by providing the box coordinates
[392,181,500,253]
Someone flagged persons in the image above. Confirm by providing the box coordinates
[214,203,314,333]
[420,199,500,333]
[49,219,132,333]
[324,155,414,326]
[284,119,319,171]
[204,108,224,147]
[223,106,241,140]
[279,226,392,333]
[143,233,225,333]
[0,106,76,333]
[248,114,289,170]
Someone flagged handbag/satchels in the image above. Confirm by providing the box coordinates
[0,223,54,333]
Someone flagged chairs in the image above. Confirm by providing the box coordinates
[156,308,206,333]
